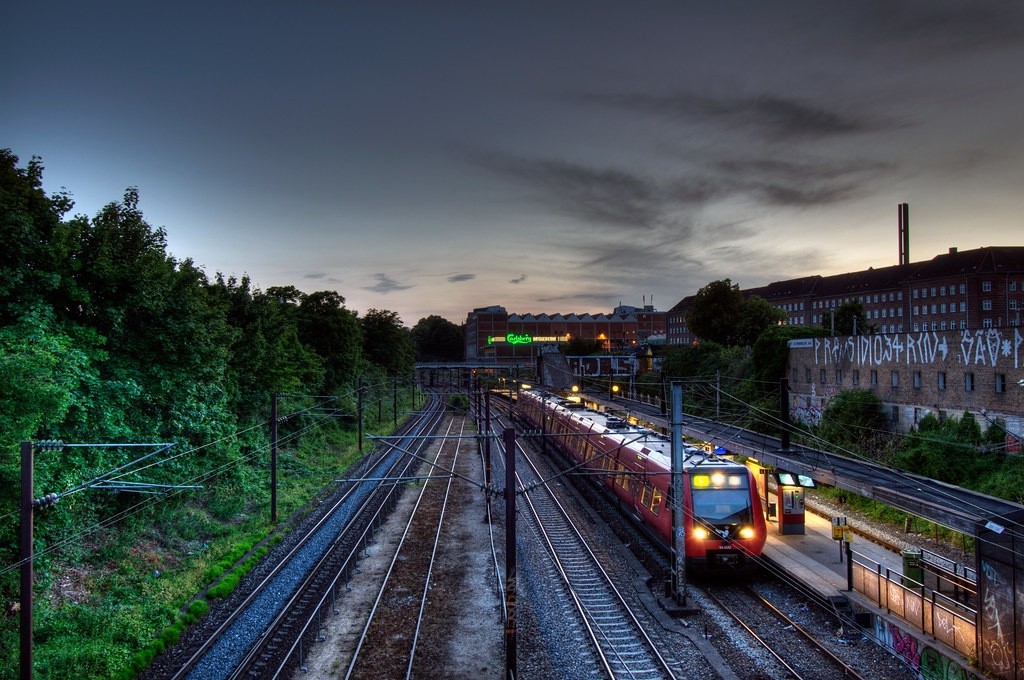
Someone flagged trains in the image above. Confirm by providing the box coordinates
[517,386,768,573]
